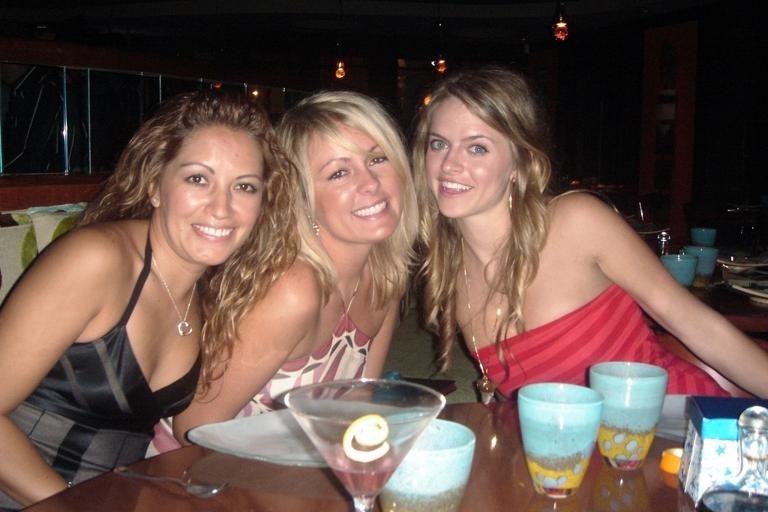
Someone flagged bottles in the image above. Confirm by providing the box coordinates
[697,406,768,511]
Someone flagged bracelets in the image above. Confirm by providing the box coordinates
[66,481,72,489]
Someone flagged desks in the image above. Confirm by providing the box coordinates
[571,169,768,349]
[28,399,768,512]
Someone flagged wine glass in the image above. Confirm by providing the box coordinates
[284,374,446,512]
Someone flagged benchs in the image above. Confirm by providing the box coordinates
[0,169,123,298]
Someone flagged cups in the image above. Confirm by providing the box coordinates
[378,415,479,509]
[586,358,670,473]
[661,227,720,287]
[511,380,604,498]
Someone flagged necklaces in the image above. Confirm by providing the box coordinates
[460,235,508,406]
[149,252,197,336]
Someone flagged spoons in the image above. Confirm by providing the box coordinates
[99,465,226,501]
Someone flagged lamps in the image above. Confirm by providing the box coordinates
[551,2,570,41]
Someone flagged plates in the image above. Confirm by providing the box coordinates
[714,252,767,306]
[183,409,336,470]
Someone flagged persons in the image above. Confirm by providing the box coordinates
[399,68,768,403]
[1,87,301,512]
[145,90,420,459]
